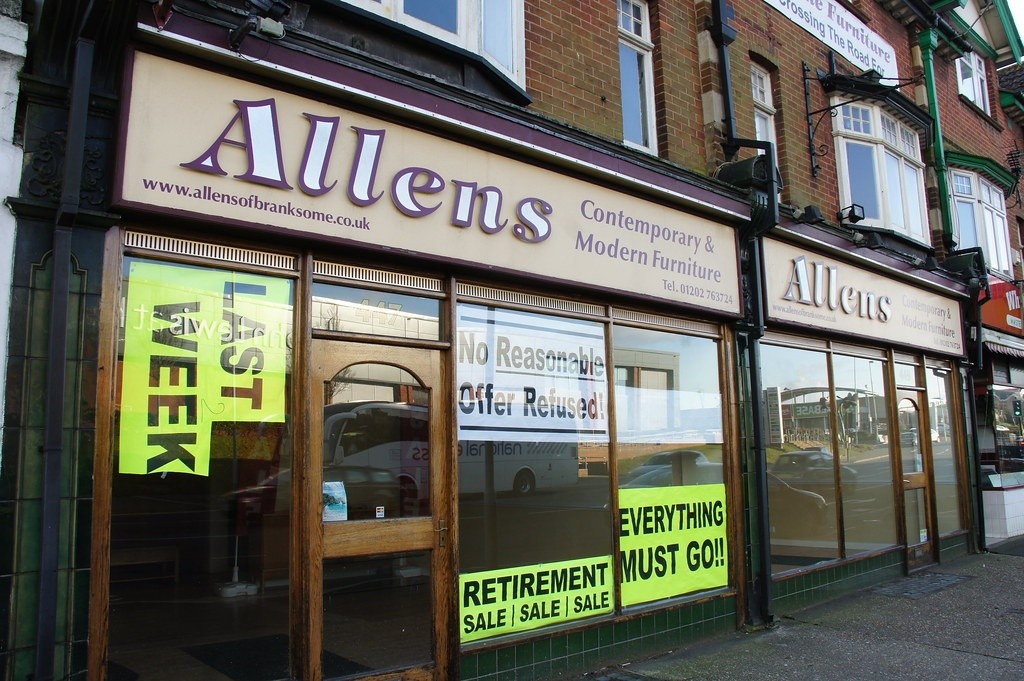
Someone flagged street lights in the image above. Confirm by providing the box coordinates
[869,360,879,444]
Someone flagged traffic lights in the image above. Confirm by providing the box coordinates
[1020,439,1024,445]
[1012,399,1024,419]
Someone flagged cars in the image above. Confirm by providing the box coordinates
[903,427,942,447]
[618,444,860,517]
[230,466,406,534]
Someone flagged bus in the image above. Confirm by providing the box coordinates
[323,403,581,501]
